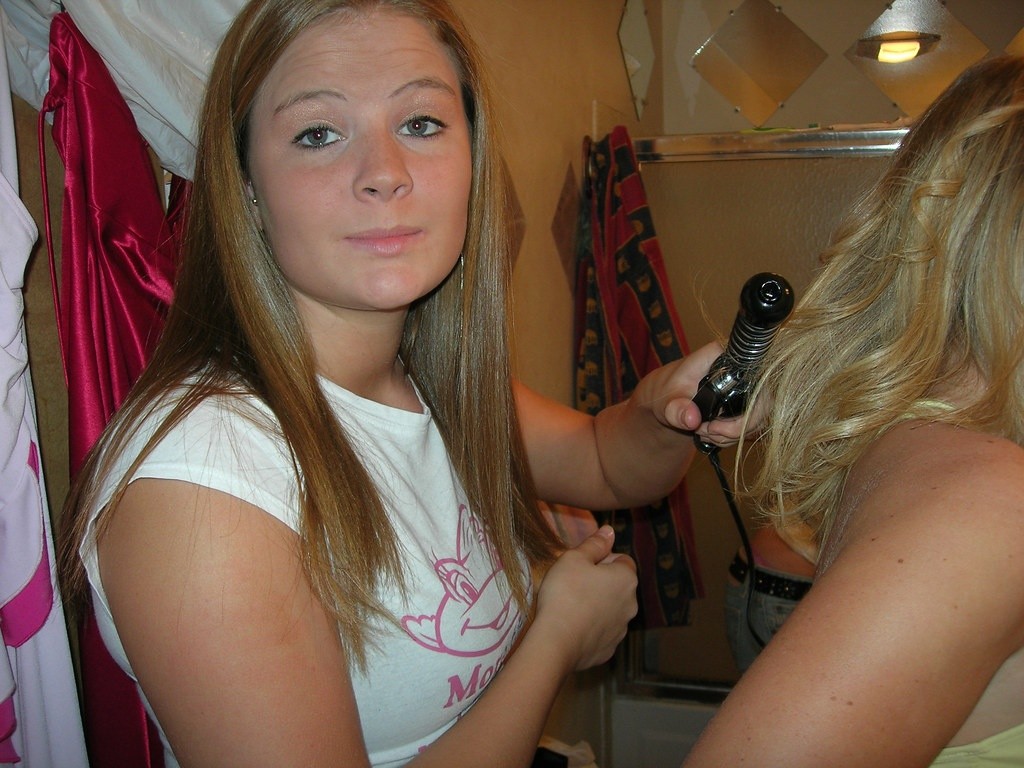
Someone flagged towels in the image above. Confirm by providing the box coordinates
[571,124,708,631]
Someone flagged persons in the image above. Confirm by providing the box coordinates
[672,45,1023,768]
[44,2,780,768]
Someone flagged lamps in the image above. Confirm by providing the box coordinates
[855,32,941,64]
